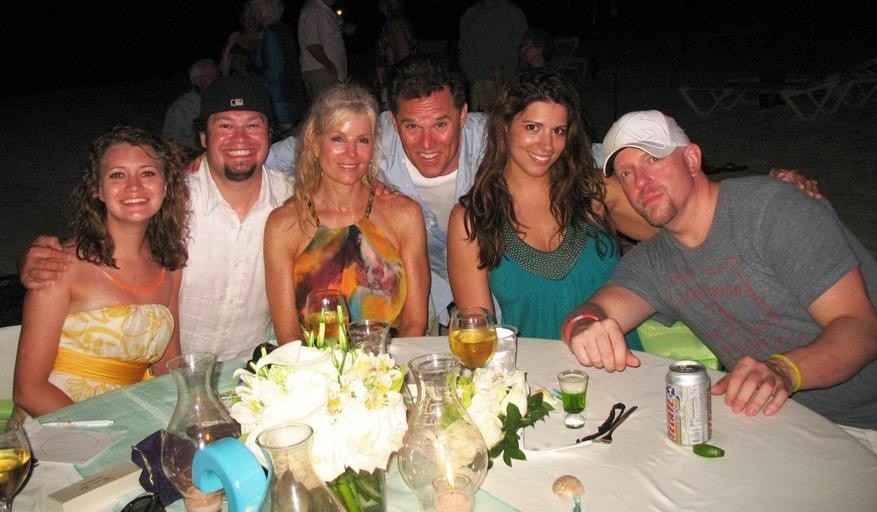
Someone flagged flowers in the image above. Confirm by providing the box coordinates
[443,362,556,469]
[228,301,409,489]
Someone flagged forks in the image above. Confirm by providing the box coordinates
[592,405,637,446]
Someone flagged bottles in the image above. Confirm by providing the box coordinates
[396,349,488,512]
[349,316,414,416]
[255,423,347,512]
[159,352,240,509]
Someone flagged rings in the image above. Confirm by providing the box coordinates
[812,180,818,184]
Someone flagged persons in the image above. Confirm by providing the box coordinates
[492,29,554,95]
[561,111,877,456]
[447,72,822,349]
[20,74,400,364]
[457,0,530,110]
[265,85,432,347]
[183,59,607,336]
[13,127,189,420]
[218,0,433,136]
[162,59,221,150]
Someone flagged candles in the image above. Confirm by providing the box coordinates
[435,440,469,511]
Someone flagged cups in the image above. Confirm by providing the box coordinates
[488,323,517,372]
[426,469,476,511]
[304,287,350,346]
[447,304,497,373]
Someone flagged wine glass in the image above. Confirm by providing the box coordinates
[558,368,588,429]
[0,418,34,511]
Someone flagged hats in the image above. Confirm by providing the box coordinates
[191,73,280,127]
[601,109,692,179]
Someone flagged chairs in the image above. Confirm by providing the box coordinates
[0,271,26,337]
[674,59,877,121]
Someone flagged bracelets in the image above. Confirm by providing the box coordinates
[222,55,232,59]
[565,315,600,346]
[771,354,802,393]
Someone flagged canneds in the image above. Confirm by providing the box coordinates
[664,359,712,447]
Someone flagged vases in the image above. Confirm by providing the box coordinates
[329,458,389,511]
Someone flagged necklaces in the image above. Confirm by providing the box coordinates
[98,267,165,292]
